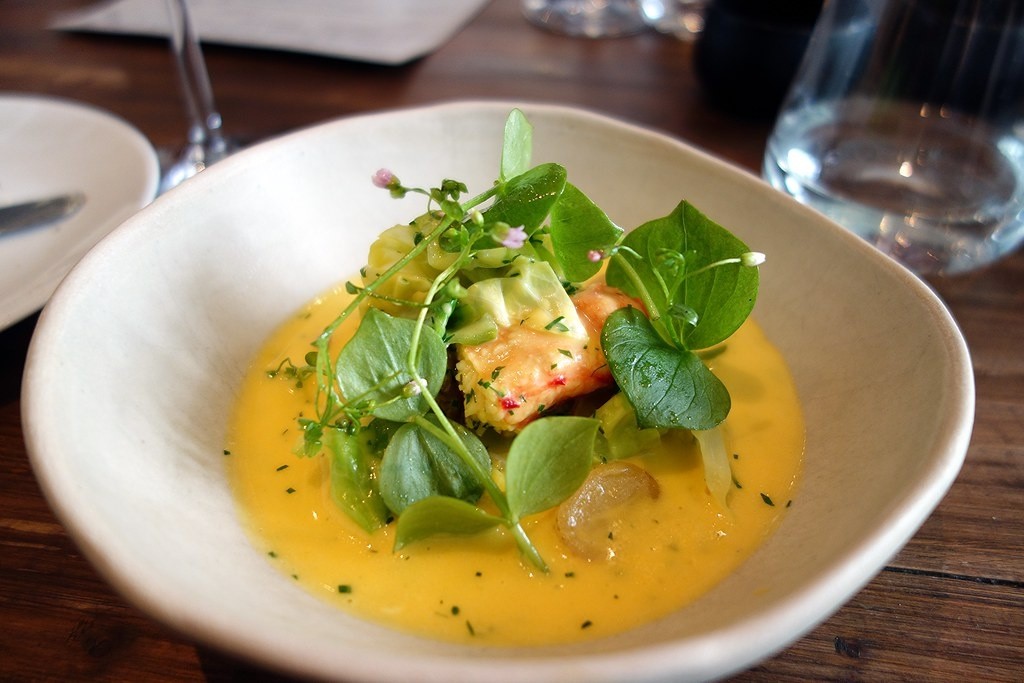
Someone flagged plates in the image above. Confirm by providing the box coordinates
[0,91,161,332]
[22,98,978,683]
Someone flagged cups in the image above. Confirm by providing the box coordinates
[757,0,1024,279]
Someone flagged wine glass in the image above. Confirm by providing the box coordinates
[157,0,245,194]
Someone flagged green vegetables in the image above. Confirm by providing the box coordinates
[266,107,765,575]
